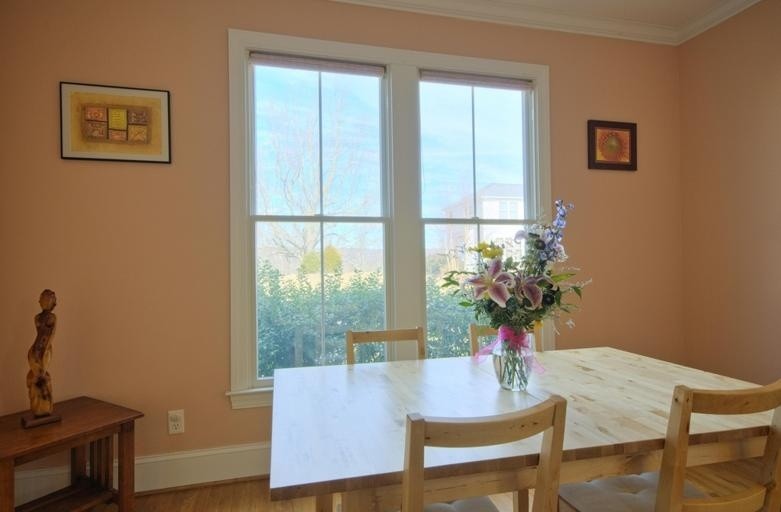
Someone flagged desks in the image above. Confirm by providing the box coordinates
[268,346,781,512]
[0,395,144,512]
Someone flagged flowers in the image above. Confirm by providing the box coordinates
[436,198,595,393]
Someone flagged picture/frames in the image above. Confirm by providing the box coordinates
[587,119,638,171]
[60,81,172,164]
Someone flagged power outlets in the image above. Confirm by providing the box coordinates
[167,409,185,434]
